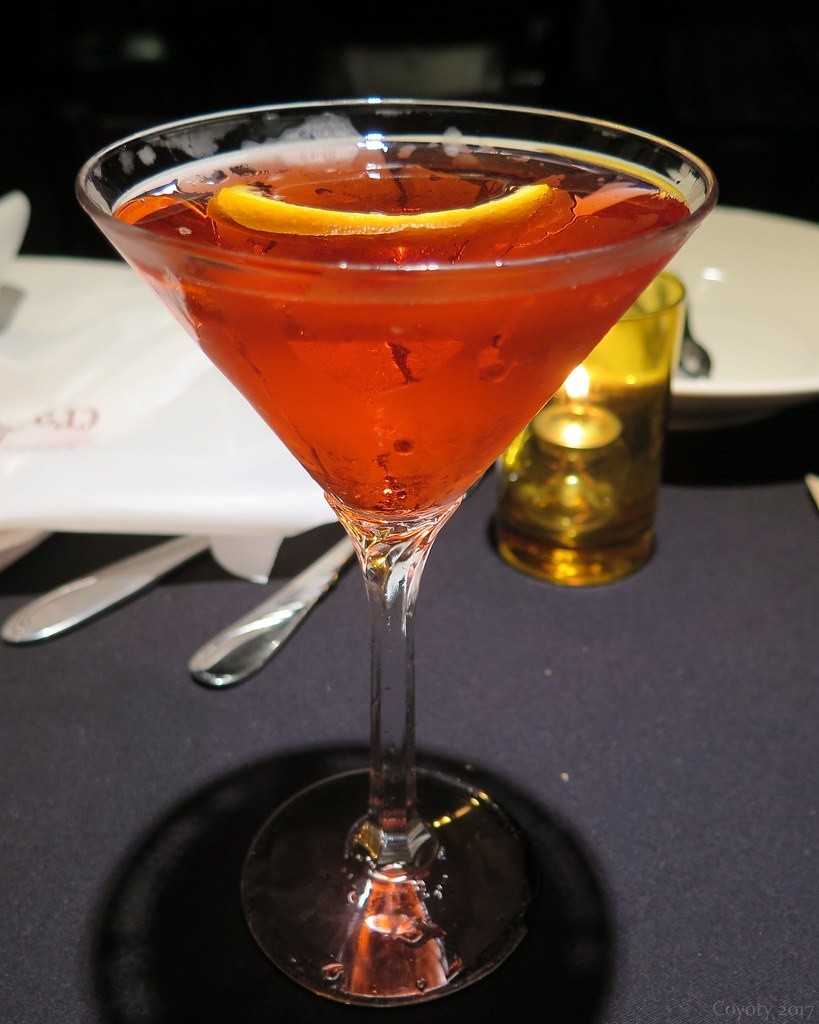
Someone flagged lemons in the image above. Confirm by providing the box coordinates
[217,184,553,237]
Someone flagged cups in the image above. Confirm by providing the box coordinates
[496,272,687,590]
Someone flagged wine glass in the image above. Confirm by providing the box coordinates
[75,98,717,1007]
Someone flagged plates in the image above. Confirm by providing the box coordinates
[665,206,819,399]
